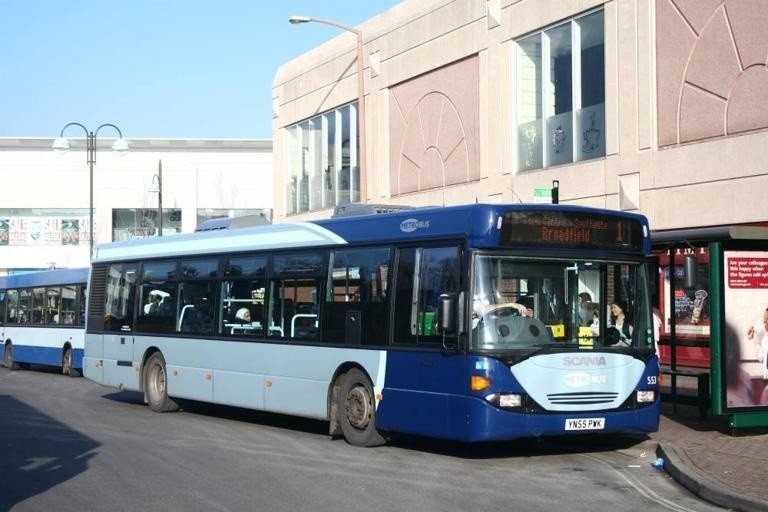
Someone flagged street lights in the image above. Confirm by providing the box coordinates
[51,122,130,266]
[289,15,368,205]
[147,159,163,236]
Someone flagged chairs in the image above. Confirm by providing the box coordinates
[149,303,213,336]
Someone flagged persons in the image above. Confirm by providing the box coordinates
[472,275,534,331]
[143,289,171,315]
[230,308,258,335]
[652,306,661,366]
[756,308,768,406]
[511,278,557,322]
[578,291,634,347]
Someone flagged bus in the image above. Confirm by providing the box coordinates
[0,265,91,378]
[82,203,666,449]
